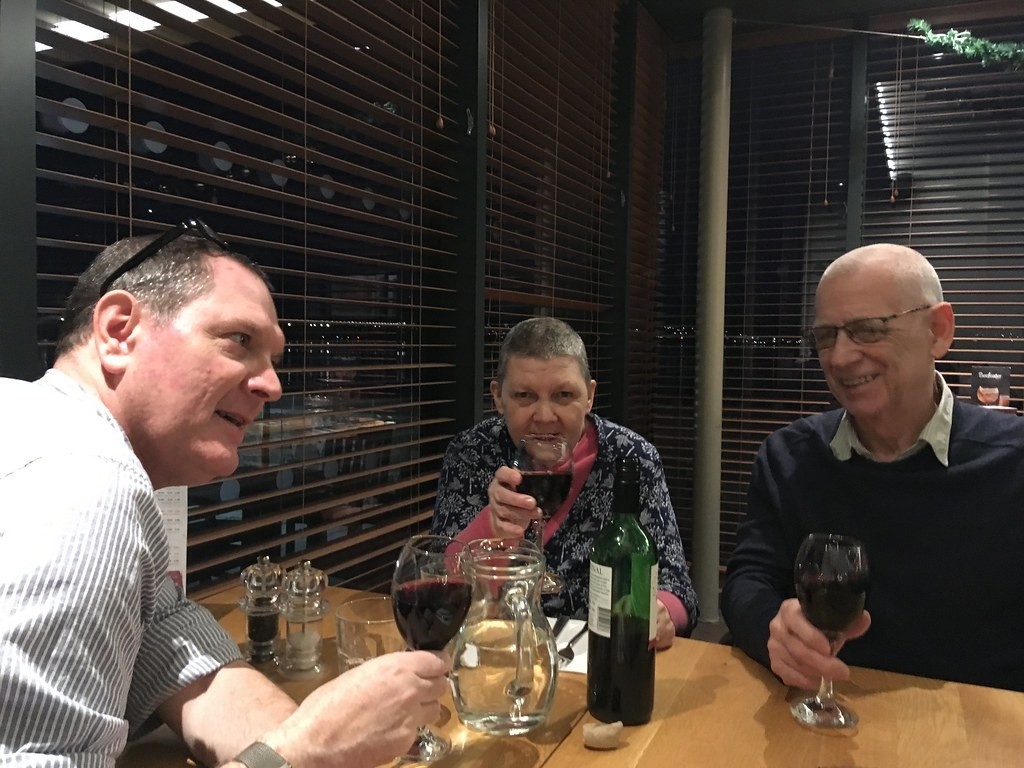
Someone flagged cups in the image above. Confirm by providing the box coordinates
[335,597,406,675]
[422,562,484,628]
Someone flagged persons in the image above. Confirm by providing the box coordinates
[1,215,454,768]
[719,244,1024,693]
[430,315,700,651]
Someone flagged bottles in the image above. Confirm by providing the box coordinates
[239,555,284,672]
[280,559,328,680]
[588,456,658,726]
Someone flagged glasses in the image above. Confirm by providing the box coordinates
[800,305,931,350]
[99,215,233,295]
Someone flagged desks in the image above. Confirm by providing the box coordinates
[113,582,1024,768]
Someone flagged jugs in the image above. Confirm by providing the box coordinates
[450,538,558,735]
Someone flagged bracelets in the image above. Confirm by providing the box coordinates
[234,742,294,768]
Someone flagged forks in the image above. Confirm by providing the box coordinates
[557,622,588,667]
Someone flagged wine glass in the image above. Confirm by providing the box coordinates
[514,435,573,594]
[791,532,868,727]
[391,536,473,763]
